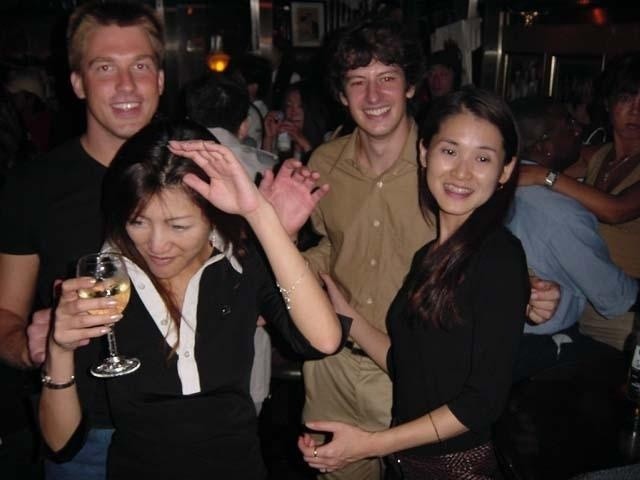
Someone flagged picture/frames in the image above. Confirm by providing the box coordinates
[290,1,327,48]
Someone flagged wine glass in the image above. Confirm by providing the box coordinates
[76,252,142,378]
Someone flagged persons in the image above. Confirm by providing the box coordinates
[1,0,639,479]
